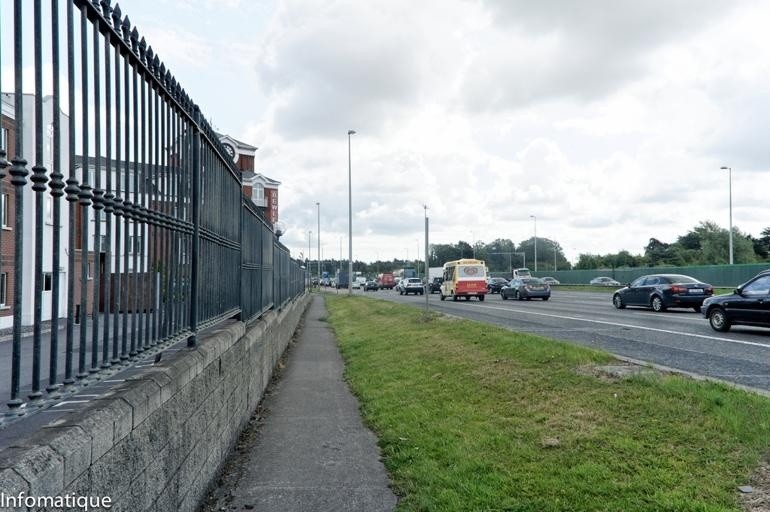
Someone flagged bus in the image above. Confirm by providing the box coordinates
[438,257,490,304]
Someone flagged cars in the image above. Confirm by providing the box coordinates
[611,273,714,313]
[310,264,444,297]
[699,268,770,334]
[486,275,510,296]
[589,276,620,288]
[540,275,560,286]
[499,276,552,301]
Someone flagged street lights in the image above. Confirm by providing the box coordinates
[530,214,539,272]
[316,202,321,293]
[720,164,734,264]
[347,129,357,296]
[308,231,313,260]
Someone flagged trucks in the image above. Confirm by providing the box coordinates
[488,267,532,282]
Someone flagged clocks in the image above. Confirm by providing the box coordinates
[222,143,235,160]
